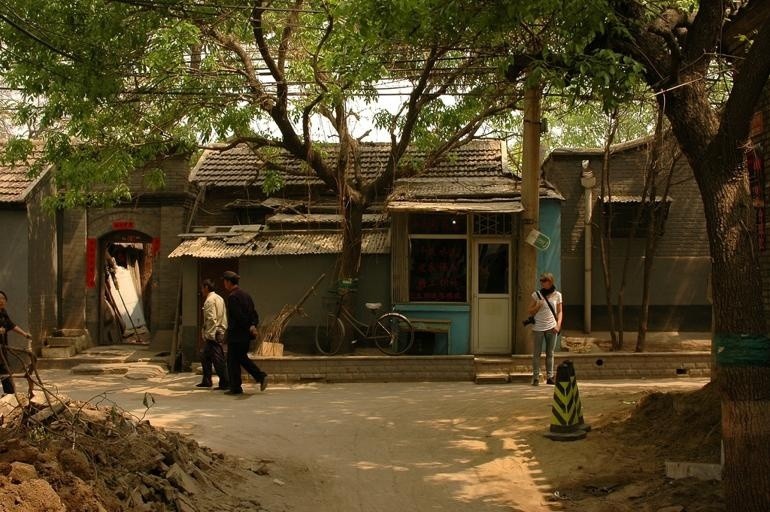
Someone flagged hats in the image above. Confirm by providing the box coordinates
[220,271,236,280]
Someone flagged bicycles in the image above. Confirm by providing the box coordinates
[314,277,415,356]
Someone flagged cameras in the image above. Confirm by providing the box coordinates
[522,316,535,326]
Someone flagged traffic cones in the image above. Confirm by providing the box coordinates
[543,359,591,440]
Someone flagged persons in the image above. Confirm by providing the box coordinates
[527,271,564,386]
[195,278,228,390]
[0,290,33,394]
[222,270,269,396]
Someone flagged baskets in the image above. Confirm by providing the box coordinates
[321,295,338,314]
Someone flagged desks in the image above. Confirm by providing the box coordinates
[393,316,453,354]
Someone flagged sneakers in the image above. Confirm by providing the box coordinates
[547,376,555,384]
[261,376,268,391]
[196,383,243,396]
[533,379,539,385]
